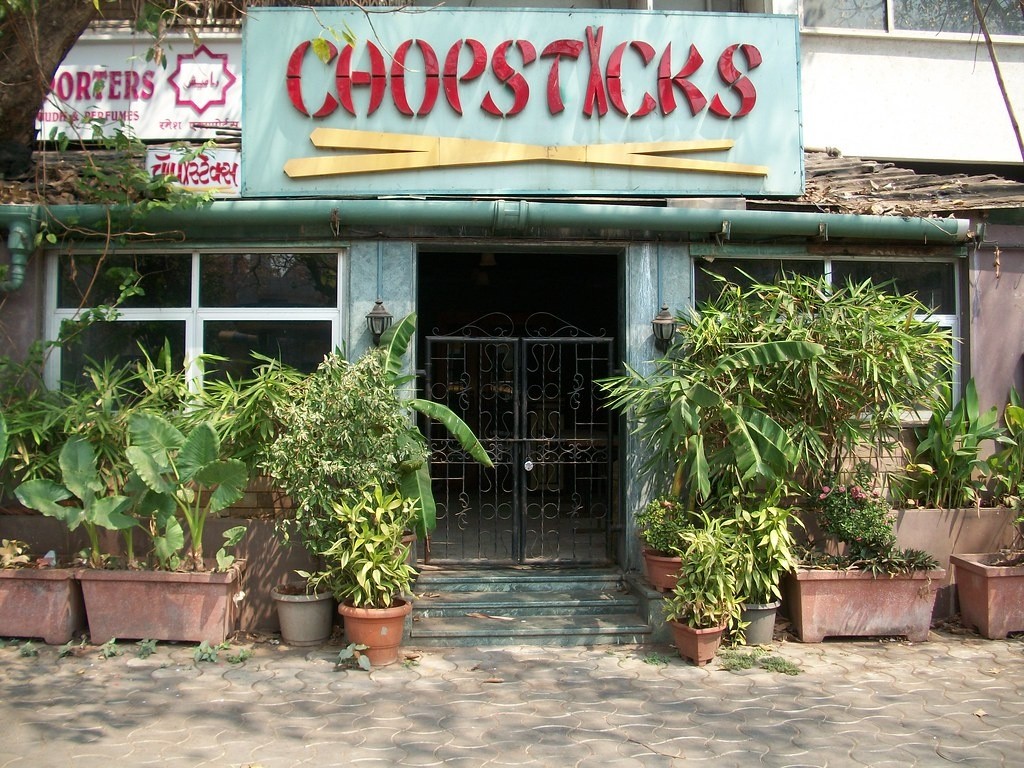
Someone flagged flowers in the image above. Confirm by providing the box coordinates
[816,486,899,560]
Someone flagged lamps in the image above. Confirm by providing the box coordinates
[652,302,678,355]
[365,300,394,346]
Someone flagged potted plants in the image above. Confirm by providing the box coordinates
[0,78,495,668]
[589,260,1024,668]
